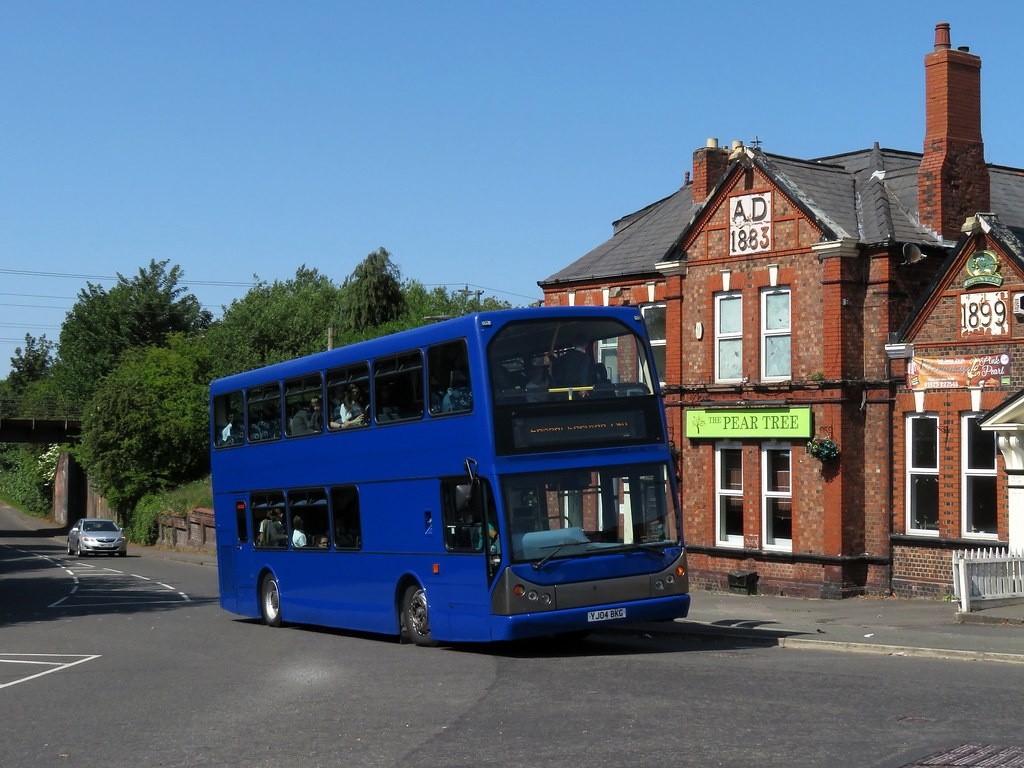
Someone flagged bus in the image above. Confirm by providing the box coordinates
[209,308,692,647]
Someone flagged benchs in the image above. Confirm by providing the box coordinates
[215,364,617,448]
[239,507,536,548]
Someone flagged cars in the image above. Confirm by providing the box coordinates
[66,518,128,557]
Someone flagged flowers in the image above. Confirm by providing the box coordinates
[807,436,839,459]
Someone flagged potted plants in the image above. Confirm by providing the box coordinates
[807,373,825,389]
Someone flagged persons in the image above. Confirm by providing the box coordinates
[217,333,597,564]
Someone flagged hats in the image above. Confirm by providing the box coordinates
[271,508,283,516]
[335,385,344,392]
